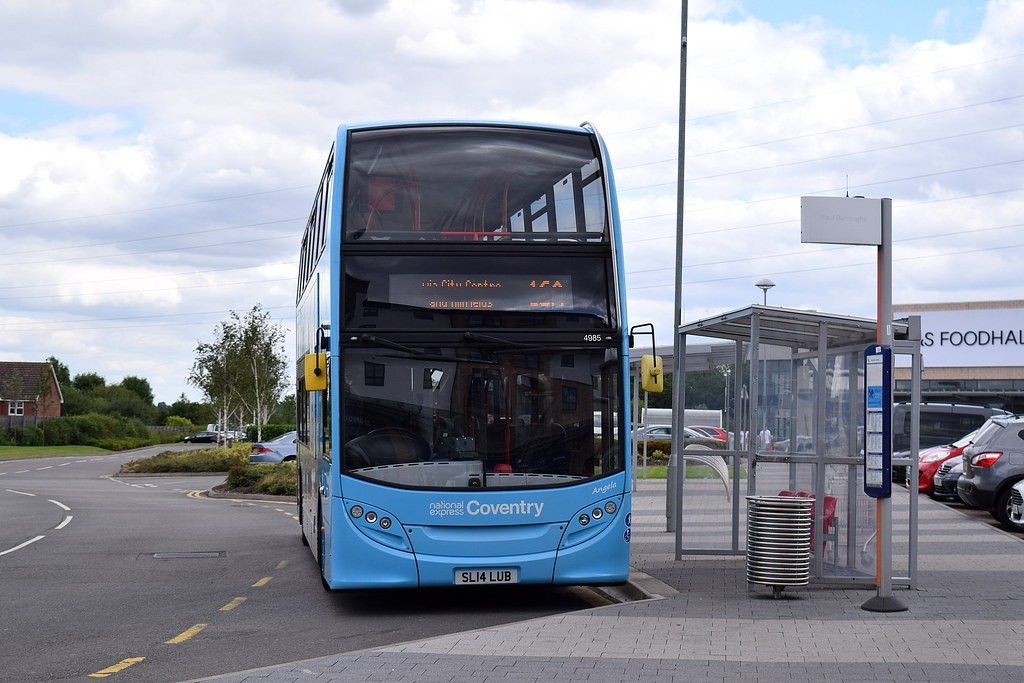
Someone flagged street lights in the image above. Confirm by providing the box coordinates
[716,363,731,450]
[754,276,776,453]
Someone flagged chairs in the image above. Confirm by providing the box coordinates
[777,490,838,576]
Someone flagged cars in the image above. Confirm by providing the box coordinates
[184,430,246,444]
[906,428,978,493]
[768,434,814,454]
[933,454,963,506]
[250,430,298,465]
[631,425,727,441]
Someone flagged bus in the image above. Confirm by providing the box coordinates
[294,120,664,592]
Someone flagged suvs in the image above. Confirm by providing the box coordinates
[957,416,1024,530]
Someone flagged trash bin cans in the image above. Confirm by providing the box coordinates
[744,492,814,588]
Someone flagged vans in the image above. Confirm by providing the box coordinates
[893,399,1015,484]
[593,410,620,440]
